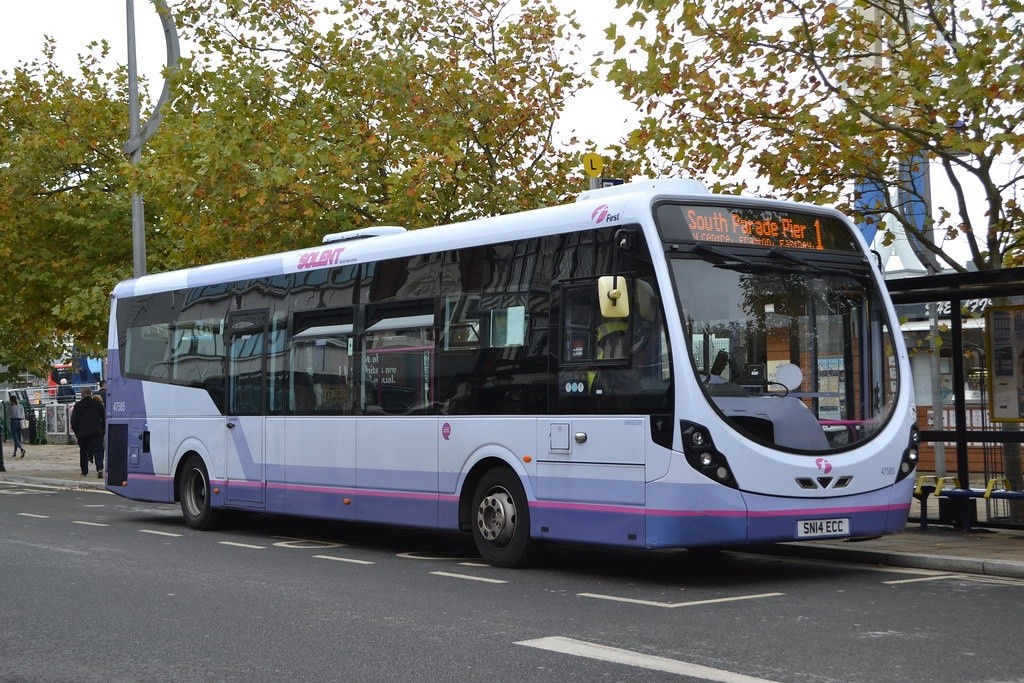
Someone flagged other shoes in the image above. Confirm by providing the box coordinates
[89,459,94,464]
[12,455,16,456]
[97,469,103,478]
[77,475,87,478]
[21,450,25,458]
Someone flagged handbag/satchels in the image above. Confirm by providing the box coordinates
[20,420,29,429]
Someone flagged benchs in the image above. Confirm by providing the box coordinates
[913,475,941,528]
[935,477,1024,532]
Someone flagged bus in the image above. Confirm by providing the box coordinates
[45,363,74,403]
[71,337,108,400]
[98,174,924,571]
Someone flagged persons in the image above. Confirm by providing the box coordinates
[7,396,25,458]
[71,379,107,478]
[234,359,569,415]
[57,378,76,404]
